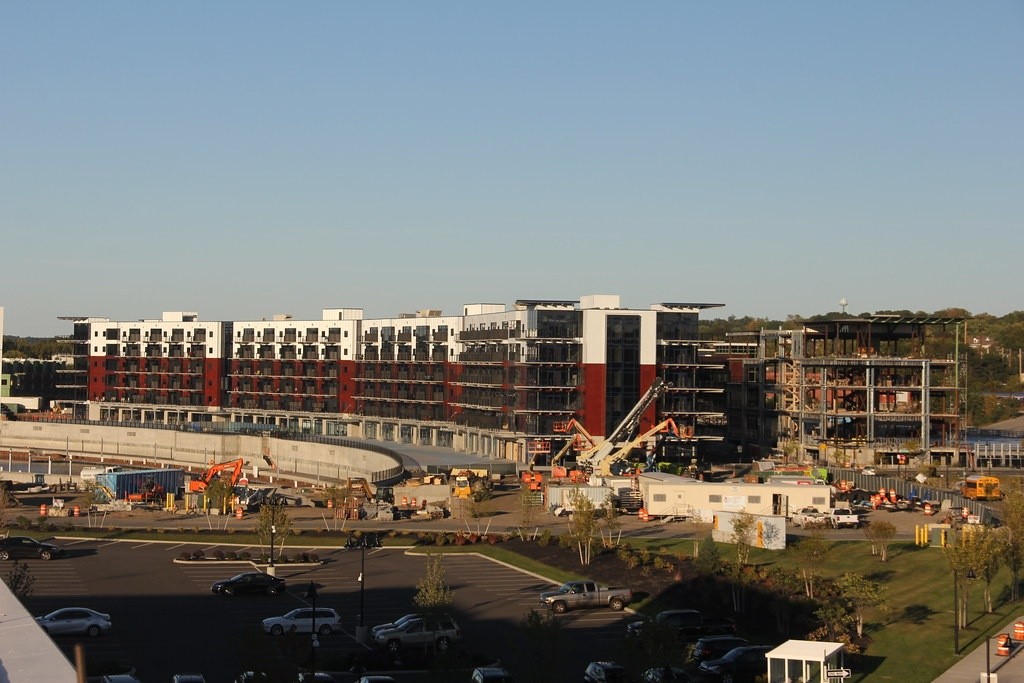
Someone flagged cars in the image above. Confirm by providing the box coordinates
[471,663,516,683]
[233,671,274,683]
[33,607,112,638]
[640,665,699,683]
[171,672,207,683]
[296,671,337,683]
[624,608,776,683]
[369,610,463,655]
[355,675,396,683]
[210,571,287,599]
[862,465,881,476]
[98,671,143,683]
[0,535,60,561]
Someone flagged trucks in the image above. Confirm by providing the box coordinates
[79,465,122,484]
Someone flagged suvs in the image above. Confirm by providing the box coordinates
[261,607,343,637]
[582,660,638,683]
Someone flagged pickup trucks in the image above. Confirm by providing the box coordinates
[539,579,633,614]
[791,505,860,529]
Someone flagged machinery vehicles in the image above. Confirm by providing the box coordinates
[343,476,395,507]
[189,457,244,495]
[552,375,695,485]
[453,473,480,499]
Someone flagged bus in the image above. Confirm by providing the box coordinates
[958,474,1001,502]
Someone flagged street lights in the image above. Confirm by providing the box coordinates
[985,633,1016,683]
[303,580,320,683]
[270,497,276,567]
